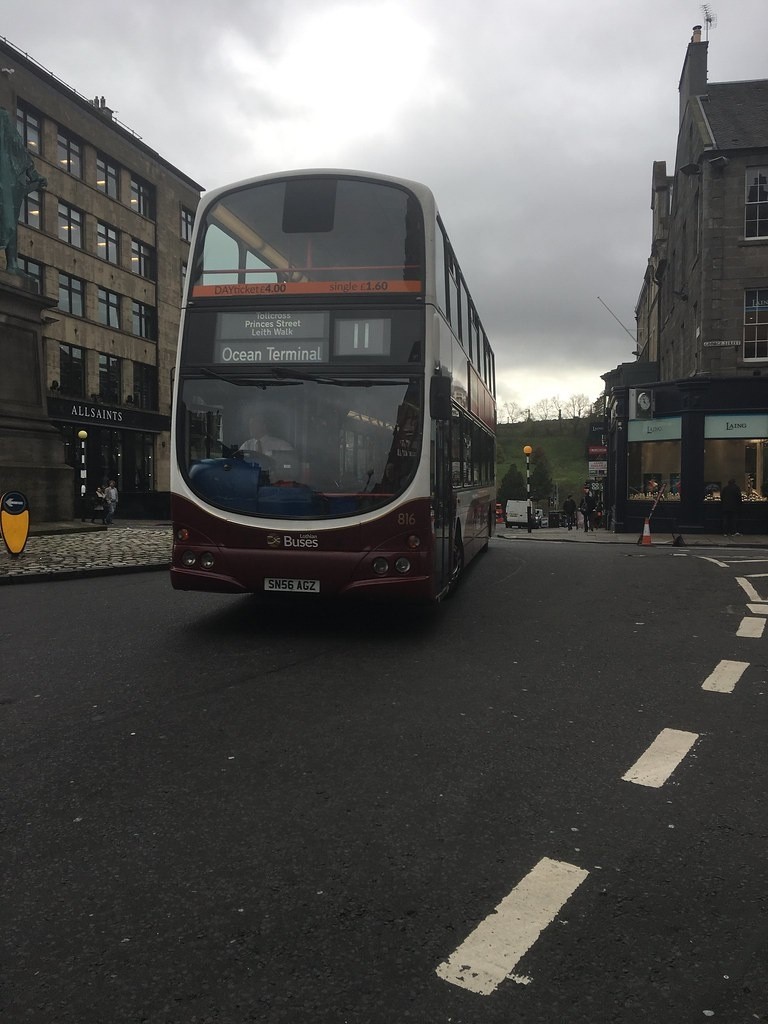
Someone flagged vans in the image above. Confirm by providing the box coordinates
[505,500,534,529]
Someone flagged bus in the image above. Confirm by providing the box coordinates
[168,167,498,610]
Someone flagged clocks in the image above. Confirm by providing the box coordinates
[638,393,651,410]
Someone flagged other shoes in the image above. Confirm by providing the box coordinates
[568,527,570,531]
[590,526,593,531]
[732,532,739,536]
[570,525,572,530]
[724,534,728,536]
[584,531,588,532]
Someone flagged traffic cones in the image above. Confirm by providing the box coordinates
[638,517,654,546]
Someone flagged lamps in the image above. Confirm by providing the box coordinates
[78,430,88,443]
[50,380,58,392]
[126,395,135,404]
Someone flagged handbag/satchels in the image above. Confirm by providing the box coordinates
[580,496,588,514]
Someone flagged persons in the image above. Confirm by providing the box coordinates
[91,486,108,525]
[579,490,596,532]
[563,493,577,531]
[720,479,742,537]
[236,413,296,471]
[104,481,118,525]
[379,462,404,497]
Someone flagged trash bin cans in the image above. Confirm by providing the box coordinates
[548,512,560,528]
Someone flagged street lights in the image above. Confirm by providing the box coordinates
[78,430,87,523]
[524,445,533,532]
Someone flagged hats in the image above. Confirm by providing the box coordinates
[728,479,736,484]
